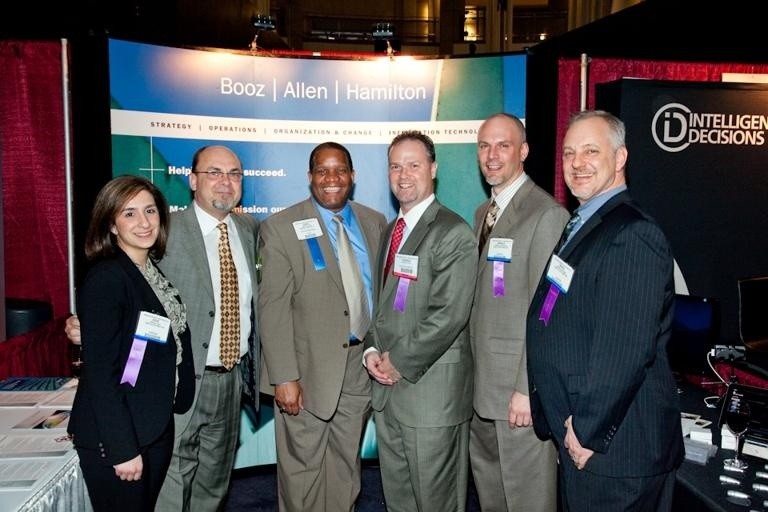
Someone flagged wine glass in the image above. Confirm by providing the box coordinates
[722,400,751,470]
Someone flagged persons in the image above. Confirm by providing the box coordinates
[64,174,196,512]
[64,144,256,512]
[362,129,479,512]
[523,109,684,512]
[472,111,569,512]
[258,141,386,512]
[606,511,608,512]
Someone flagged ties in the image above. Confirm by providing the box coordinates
[332,214,371,341]
[382,218,406,289]
[557,212,580,256]
[217,223,240,371]
[478,202,500,259]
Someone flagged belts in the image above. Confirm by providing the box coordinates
[206,353,248,373]
[349,338,365,346]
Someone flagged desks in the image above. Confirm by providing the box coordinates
[674,374,768,512]
[0,375,93,512]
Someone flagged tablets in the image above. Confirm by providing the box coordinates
[717,384,768,448]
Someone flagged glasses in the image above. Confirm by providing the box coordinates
[195,172,243,182]
